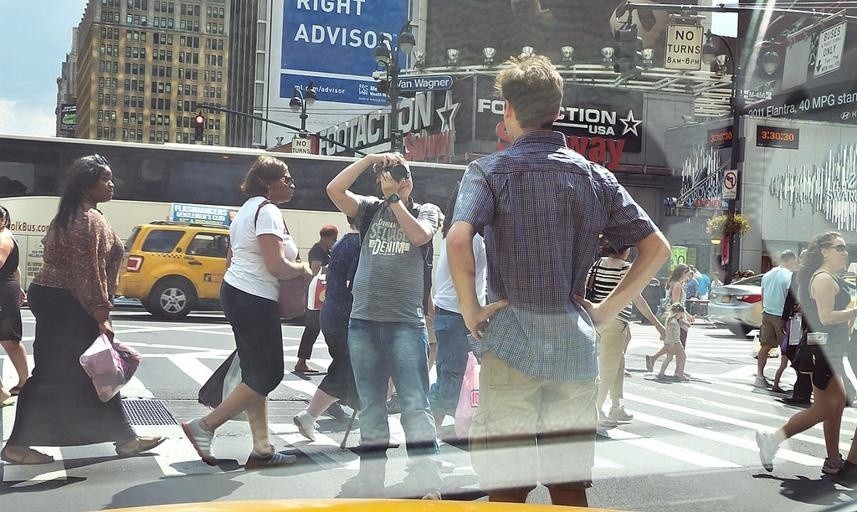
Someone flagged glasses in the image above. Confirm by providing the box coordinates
[94,154,108,163]
[830,244,847,252]
[282,176,294,183]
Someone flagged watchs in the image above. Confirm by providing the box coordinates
[387,193,400,203]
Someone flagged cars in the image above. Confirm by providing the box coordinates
[707,272,765,336]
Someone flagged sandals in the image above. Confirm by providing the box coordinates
[0,442,55,464]
[113,429,166,458]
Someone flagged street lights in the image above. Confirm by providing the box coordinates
[373,19,419,152]
[702,29,783,285]
[288,80,317,139]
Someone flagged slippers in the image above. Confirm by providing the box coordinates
[0,397,16,408]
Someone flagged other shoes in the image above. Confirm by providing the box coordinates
[8,386,22,396]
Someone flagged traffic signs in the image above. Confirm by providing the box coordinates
[813,19,848,79]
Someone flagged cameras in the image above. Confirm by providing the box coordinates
[384,161,410,182]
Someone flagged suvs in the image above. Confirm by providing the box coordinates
[114,219,230,319]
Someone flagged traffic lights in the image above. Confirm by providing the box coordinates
[613,27,635,73]
[195,115,204,141]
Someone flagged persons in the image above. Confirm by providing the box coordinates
[445,54,670,505]
[295,215,358,440]
[386,178,489,431]
[761,255,772,274]
[684,263,723,316]
[646,263,696,383]
[586,238,668,426]
[0,206,28,407]
[730,269,754,286]
[0,156,167,465]
[181,155,313,470]
[325,151,440,498]
[751,232,856,482]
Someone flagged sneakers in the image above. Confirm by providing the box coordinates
[754,377,857,475]
[609,405,633,421]
[246,444,298,469]
[646,355,689,380]
[598,410,617,427]
[182,417,218,466]
[293,410,320,442]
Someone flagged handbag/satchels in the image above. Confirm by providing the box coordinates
[222,351,249,421]
[79,334,143,403]
[655,295,672,327]
[197,348,238,410]
[277,276,306,320]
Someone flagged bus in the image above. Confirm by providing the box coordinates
[0,133,467,295]
